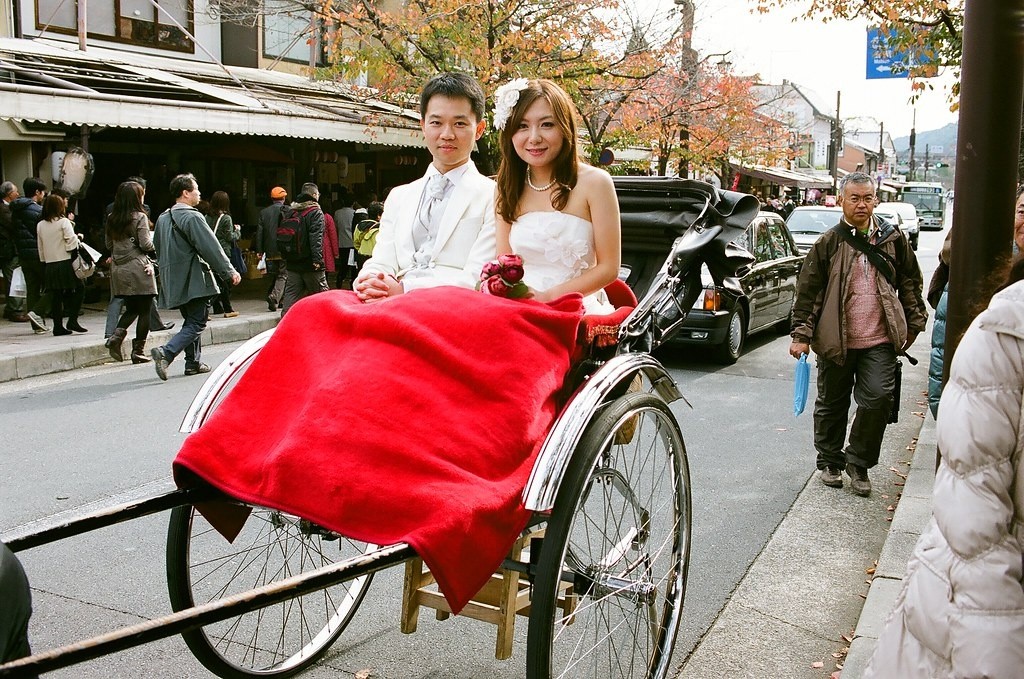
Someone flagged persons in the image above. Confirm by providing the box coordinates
[494,80,622,315]
[862,182,1024,679]
[0,172,392,381]
[756,192,843,220]
[353,73,499,302]
[789,173,927,497]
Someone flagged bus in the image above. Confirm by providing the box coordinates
[900,181,946,230]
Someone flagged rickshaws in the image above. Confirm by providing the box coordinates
[0,176,760,679]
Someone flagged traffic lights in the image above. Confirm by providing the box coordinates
[936,163,949,168]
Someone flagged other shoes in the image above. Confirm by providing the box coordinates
[207,315,211,321]
[278,302,283,308]
[4,311,30,322]
[266,297,276,311]
[105,332,111,338]
[336,274,342,289]
[224,310,239,318]
[151,322,175,331]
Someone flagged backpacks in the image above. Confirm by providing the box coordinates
[276,206,319,255]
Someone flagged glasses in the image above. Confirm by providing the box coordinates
[843,195,877,205]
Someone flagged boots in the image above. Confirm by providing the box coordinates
[53,313,72,336]
[131,338,151,364]
[66,304,87,332]
[105,328,127,362]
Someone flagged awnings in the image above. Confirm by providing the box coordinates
[728,162,831,189]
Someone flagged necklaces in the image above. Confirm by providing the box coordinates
[527,164,556,191]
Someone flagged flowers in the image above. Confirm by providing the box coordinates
[492,77,529,130]
[475,254,535,300]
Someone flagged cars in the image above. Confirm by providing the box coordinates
[784,196,844,255]
[665,211,809,361]
[872,202,924,251]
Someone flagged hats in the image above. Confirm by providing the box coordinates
[270,187,288,198]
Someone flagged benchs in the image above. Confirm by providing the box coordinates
[559,279,638,405]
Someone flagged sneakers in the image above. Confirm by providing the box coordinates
[27,311,47,330]
[151,346,169,381]
[846,463,871,494]
[184,362,212,376]
[34,326,53,334]
[821,466,843,487]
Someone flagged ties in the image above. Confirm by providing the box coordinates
[420,174,448,230]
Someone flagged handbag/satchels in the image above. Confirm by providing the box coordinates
[232,238,246,275]
[9,266,27,298]
[71,239,102,279]
[256,253,268,275]
[793,353,810,417]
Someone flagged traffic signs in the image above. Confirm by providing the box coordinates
[866,26,939,80]
